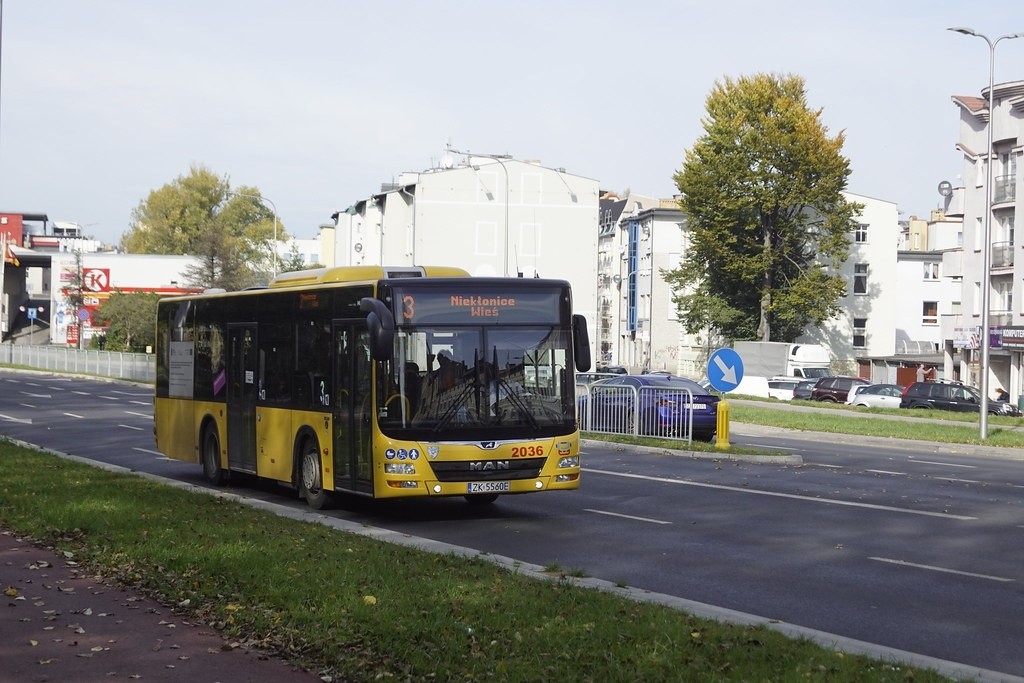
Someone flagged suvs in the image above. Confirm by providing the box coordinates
[898,381,1022,416]
[768,375,876,405]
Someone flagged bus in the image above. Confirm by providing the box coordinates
[154,263,592,511]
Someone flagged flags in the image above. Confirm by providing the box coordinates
[4,242,20,267]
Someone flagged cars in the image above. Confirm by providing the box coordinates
[530,360,720,443]
[844,384,905,409]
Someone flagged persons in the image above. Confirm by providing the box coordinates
[916,364,932,382]
[604,350,612,363]
[422,348,461,397]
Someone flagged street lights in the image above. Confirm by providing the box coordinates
[457,151,508,278]
[227,192,276,284]
[946,25,1024,442]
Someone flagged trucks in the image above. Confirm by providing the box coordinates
[733,341,835,379]
[698,376,769,401]
